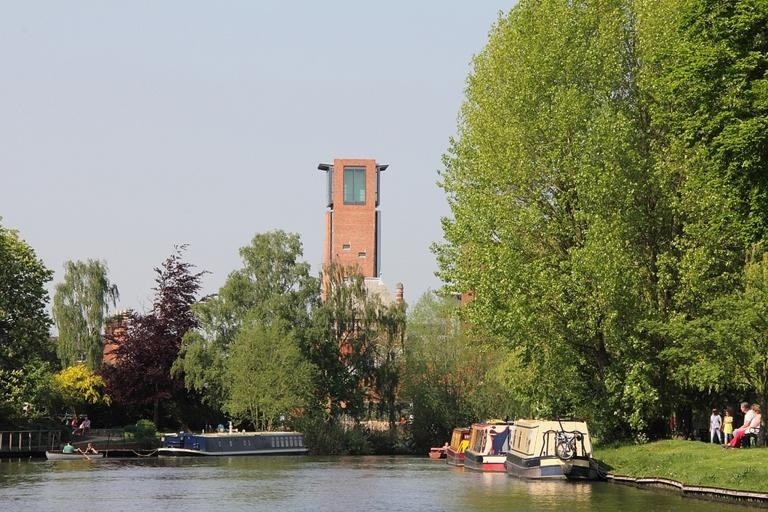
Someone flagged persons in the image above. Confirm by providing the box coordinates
[731,402,752,437]
[81,417,90,437]
[85,443,97,455]
[707,408,722,444]
[719,403,761,449]
[62,440,74,454]
[720,409,733,444]
[71,416,78,435]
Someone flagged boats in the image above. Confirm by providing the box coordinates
[44,421,309,462]
[428,419,599,480]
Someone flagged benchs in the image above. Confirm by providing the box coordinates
[744,427,759,448]
